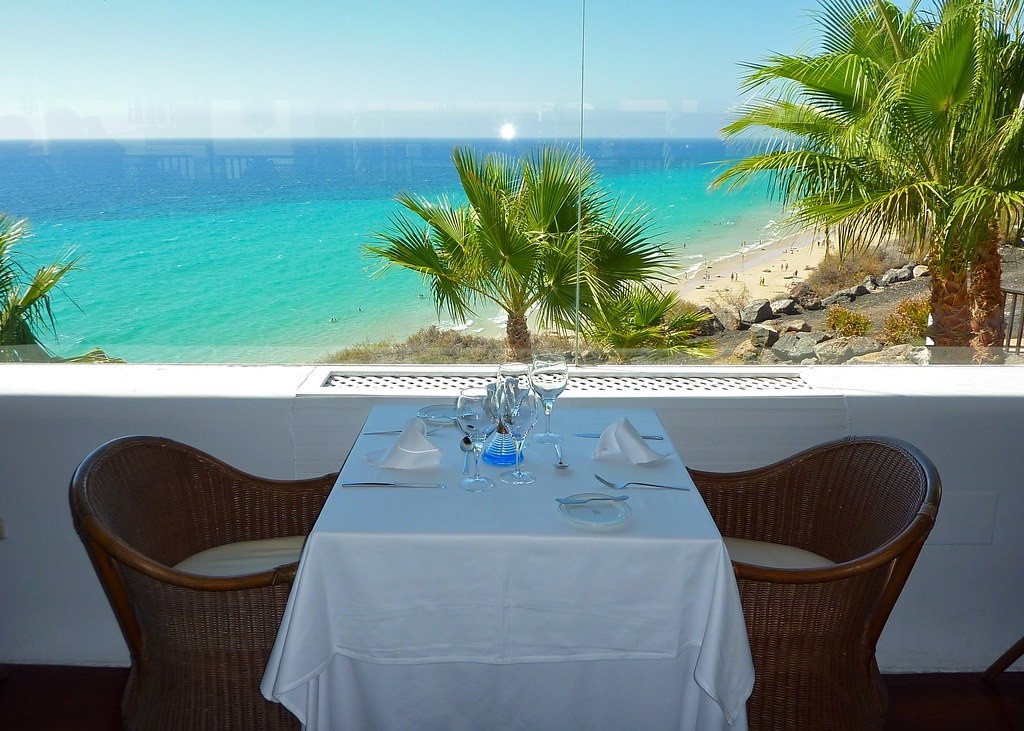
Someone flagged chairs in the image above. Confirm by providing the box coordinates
[685,435,943,731]
[69,435,339,731]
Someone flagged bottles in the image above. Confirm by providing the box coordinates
[481,423,523,466]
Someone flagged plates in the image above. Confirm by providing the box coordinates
[559,493,632,527]
[416,404,472,423]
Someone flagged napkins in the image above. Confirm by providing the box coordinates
[592,417,671,464]
[365,416,440,469]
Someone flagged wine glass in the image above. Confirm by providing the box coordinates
[500,387,537,485]
[530,355,568,444]
[457,387,499,493]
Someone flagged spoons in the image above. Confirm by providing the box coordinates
[417,415,457,420]
[460,435,474,474]
[364,425,444,435]
[554,445,568,468]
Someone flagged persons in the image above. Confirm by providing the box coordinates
[818,242,820,246]
[660,284,662,290]
[759,276,765,286]
[683,243,686,248]
[708,272,710,279]
[730,272,734,281]
[706,271,707,278]
[684,272,687,280]
[781,263,783,270]
[785,263,788,269]
[795,269,798,278]
[735,273,738,281]
[705,258,707,265]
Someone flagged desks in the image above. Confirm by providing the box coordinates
[260,405,755,731]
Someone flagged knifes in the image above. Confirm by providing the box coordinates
[576,430,663,439]
[342,481,445,488]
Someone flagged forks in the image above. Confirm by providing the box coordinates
[594,474,690,490]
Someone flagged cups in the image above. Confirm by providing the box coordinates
[498,363,531,416]
